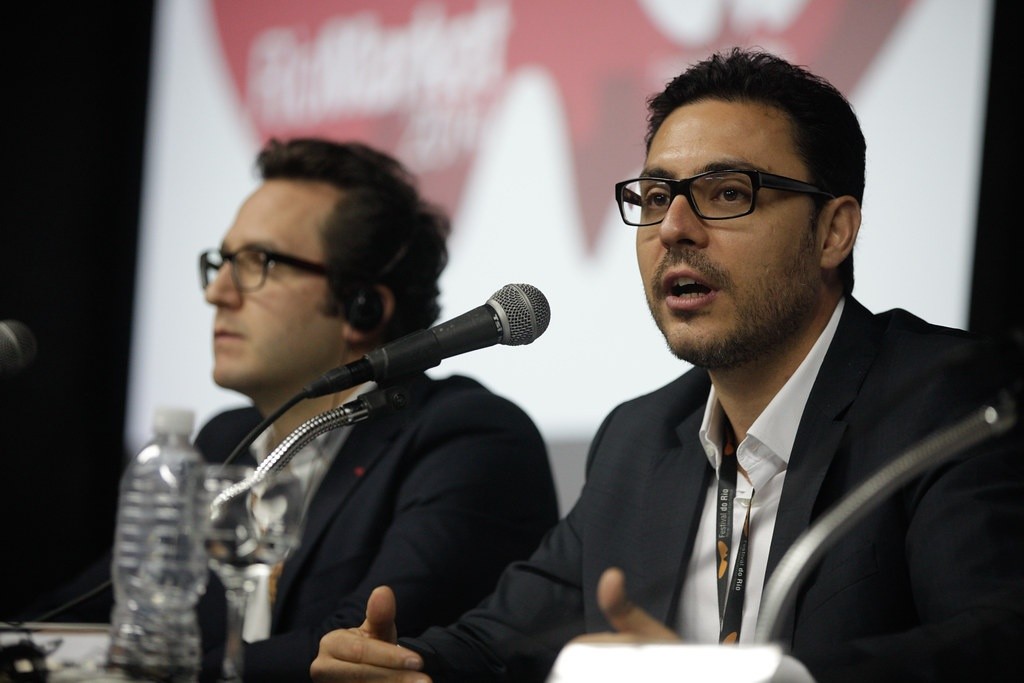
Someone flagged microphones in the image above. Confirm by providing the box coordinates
[304,283,550,400]
[0,319,37,373]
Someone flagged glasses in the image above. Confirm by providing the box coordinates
[615,168,837,226]
[200,245,332,292]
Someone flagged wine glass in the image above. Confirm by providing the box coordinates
[181,463,302,683]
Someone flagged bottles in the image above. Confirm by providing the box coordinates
[103,405,212,682]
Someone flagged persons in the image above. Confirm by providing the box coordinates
[307,43,1024,682]
[190,138,562,683]
